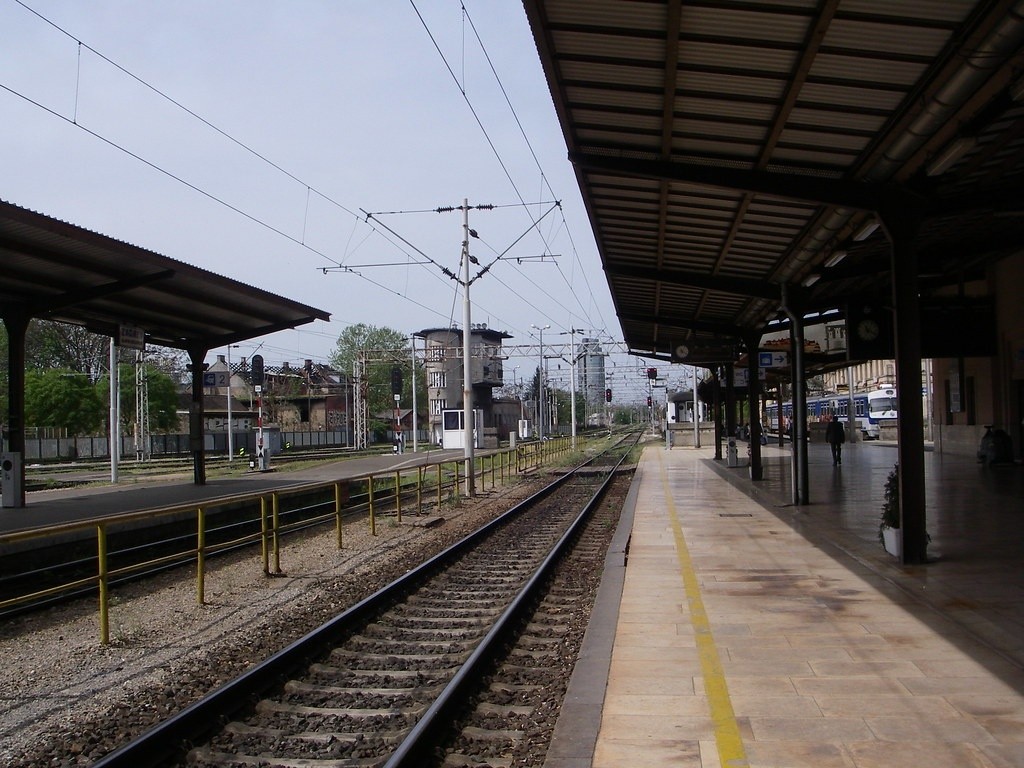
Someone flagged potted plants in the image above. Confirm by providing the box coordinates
[879,463,932,558]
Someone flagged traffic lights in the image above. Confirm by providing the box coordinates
[647,397,651,406]
[606,389,612,402]
[391,367,404,394]
[251,354,265,385]
[647,367,658,379]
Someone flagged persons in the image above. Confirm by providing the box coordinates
[825,416,846,466]
[760,423,767,446]
[735,422,750,440]
[787,416,793,445]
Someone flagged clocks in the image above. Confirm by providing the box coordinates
[857,320,879,341]
[675,345,688,359]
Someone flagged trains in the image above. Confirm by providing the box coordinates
[760,382,898,440]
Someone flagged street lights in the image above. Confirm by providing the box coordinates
[530,324,550,443]
